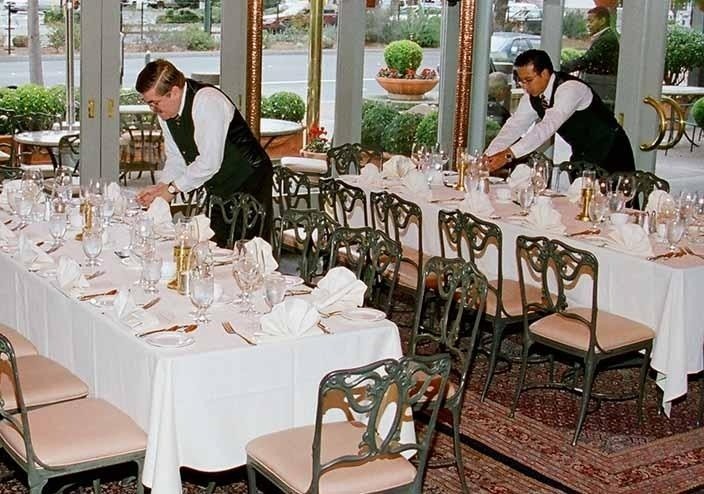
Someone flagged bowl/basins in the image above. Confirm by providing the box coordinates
[495,188,512,201]
[610,213,629,225]
[534,196,552,206]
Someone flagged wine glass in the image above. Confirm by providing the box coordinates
[82,232,103,266]
[28,167,44,202]
[232,265,255,306]
[594,179,612,207]
[533,160,548,197]
[589,200,606,228]
[7,185,21,216]
[50,186,72,223]
[90,178,106,205]
[658,192,679,243]
[616,176,636,212]
[232,240,257,298]
[432,144,449,172]
[691,197,704,232]
[464,176,478,192]
[518,184,534,216]
[82,205,103,232]
[15,191,32,228]
[80,184,95,204]
[130,226,155,286]
[21,173,40,208]
[238,249,265,315]
[175,217,199,249]
[264,275,287,315]
[100,196,114,226]
[47,213,66,248]
[189,272,215,324]
[411,143,426,170]
[666,219,685,251]
[678,191,699,237]
[142,255,162,293]
[54,168,73,187]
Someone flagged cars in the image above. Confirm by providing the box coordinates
[262,3,337,33]
[509,4,543,33]
[489,32,541,74]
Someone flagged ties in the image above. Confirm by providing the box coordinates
[173,115,180,127]
[541,95,548,110]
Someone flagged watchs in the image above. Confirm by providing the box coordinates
[168,181,179,196]
[504,151,513,162]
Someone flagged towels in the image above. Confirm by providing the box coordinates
[569,176,600,201]
[604,222,655,259]
[105,180,121,202]
[17,232,54,272]
[0,220,13,248]
[644,188,674,241]
[261,297,321,339]
[111,286,140,320]
[458,190,495,221]
[55,255,89,292]
[357,162,380,189]
[506,163,533,191]
[382,155,415,177]
[520,200,566,236]
[147,195,173,225]
[311,265,368,316]
[195,213,214,244]
[244,235,278,278]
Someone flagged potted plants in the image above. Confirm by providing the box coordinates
[376,37,439,101]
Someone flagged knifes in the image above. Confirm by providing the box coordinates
[143,298,161,309]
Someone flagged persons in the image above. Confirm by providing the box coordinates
[477,50,642,212]
[134,59,273,251]
[560,7,620,113]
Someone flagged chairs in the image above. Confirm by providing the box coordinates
[169,182,208,218]
[437,209,562,404]
[0,358,88,421]
[368,191,440,333]
[508,150,554,190]
[324,227,404,321]
[0,108,17,161]
[556,162,607,192]
[317,177,378,303]
[0,335,147,494]
[247,353,453,494]
[9,112,63,178]
[510,235,655,447]
[59,134,81,175]
[275,208,342,280]
[117,104,160,186]
[208,193,266,250]
[271,166,321,279]
[0,321,38,362]
[605,171,671,211]
[395,256,488,493]
[333,178,414,354]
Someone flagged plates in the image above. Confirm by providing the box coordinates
[342,307,387,322]
[283,274,304,287]
[90,296,118,308]
[212,249,235,261]
[145,332,196,349]
[154,222,175,239]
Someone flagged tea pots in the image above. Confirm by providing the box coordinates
[127,214,155,263]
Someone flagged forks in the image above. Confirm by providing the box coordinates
[221,320,257,346]
[684,247,704,260]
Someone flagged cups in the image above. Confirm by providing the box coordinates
[190,241,213,272]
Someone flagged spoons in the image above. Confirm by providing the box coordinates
[81,289,117,297]
[140,325,179,336]
[649,251,674,260]
